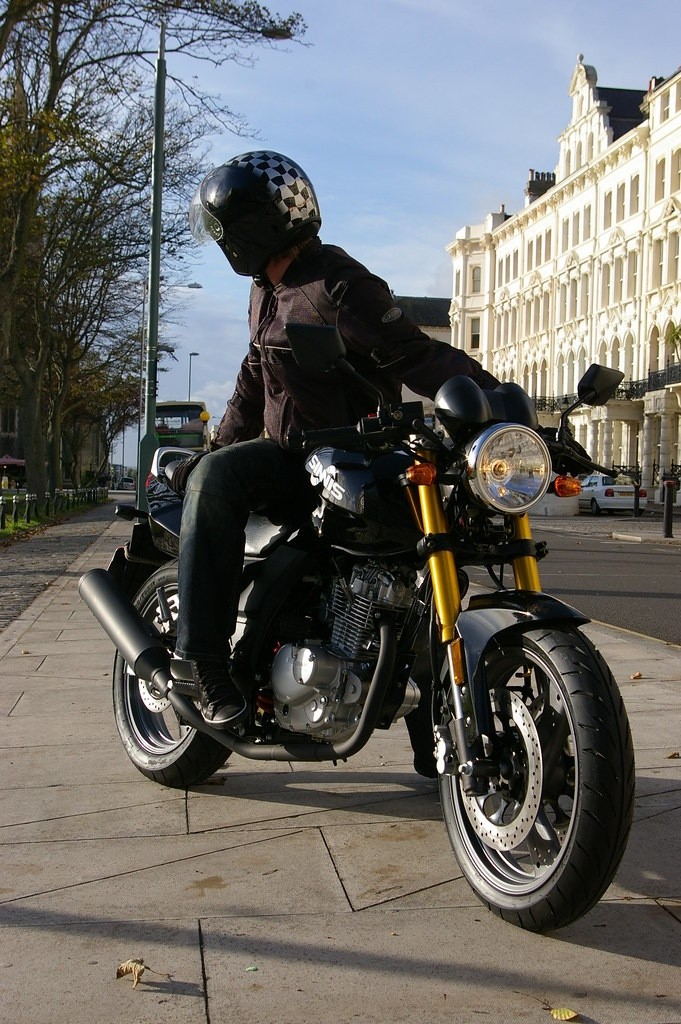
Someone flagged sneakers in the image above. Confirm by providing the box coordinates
[190,657,248,730]
[415,749,438,778]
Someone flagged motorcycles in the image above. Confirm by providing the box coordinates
[77,363,636,934]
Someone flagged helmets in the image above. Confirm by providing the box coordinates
[191,151,322,276]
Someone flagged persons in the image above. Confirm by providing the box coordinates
[171,152,592,776]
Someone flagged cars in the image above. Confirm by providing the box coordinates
[578,474,648,516]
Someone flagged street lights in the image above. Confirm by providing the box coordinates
[187,352,200,401]
[135,281,202,510]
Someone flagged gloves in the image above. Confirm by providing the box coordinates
[535,424,594,478]
[170,442,222,492]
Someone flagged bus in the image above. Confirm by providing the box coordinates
[155,400,215,453]
